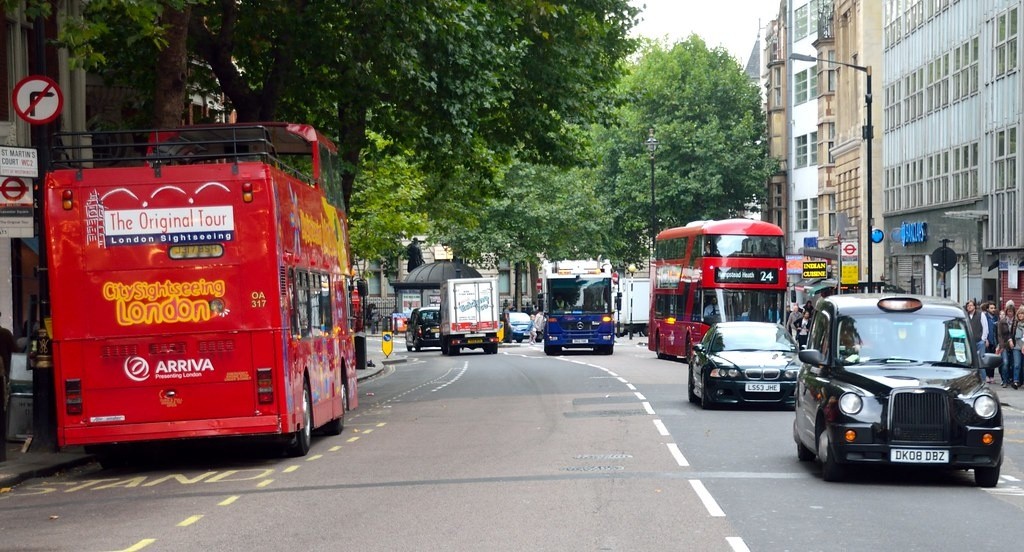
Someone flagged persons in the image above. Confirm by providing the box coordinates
[786,301,815,350]
[966,299,1024,389]
[372,306,380,333]
[704,297,721,318]
[0,310,12,462]
[502,297,545,347]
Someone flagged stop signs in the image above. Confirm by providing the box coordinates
[536,279,543,291]
[611,271,619,284]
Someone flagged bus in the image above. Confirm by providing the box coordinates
[650,219,789,365]
[43,120,356,458]
[349,287,362,332]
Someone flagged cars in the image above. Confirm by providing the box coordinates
[403,307,443,352]
[687,321,805,411]
[791,291,1004,489]
[496,310,533,342]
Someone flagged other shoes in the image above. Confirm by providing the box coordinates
[1001,378,1021,390]
[986,376,996,384]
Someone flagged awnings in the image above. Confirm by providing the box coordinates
[793,276,837,296]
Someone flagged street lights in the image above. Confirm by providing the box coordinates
[645,128,659,255]
[790,52,876,294]
[626,263,634,341]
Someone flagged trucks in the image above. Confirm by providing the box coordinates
[612,276,654,336]
[542,273,620,356]
[442,276,501,355]
